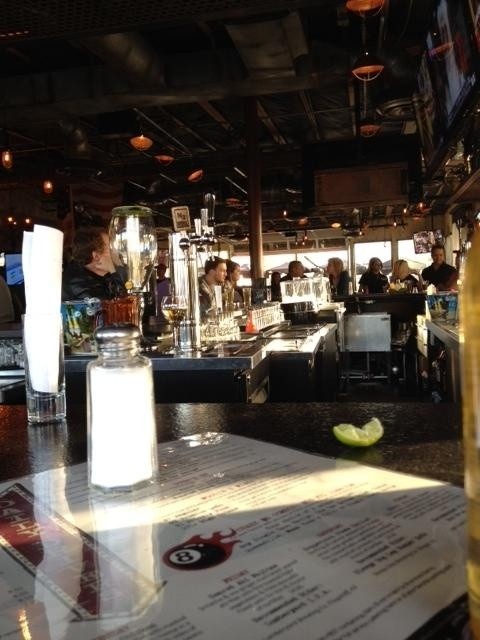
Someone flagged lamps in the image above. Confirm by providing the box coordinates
[129,107,204,185]
[2,129,56,198]
[347,2,395,152]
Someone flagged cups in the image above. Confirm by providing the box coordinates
[359,279,422,294]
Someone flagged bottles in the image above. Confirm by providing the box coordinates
[447,295,456,325]
[276,276,332,307]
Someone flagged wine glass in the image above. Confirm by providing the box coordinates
[108,206,157,353]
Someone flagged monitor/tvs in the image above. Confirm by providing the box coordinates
[410,0,480,183]
[0,251,24,286]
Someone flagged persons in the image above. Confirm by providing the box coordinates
[272,272,280,289]
[0,273,22,326]
[421,244,458,292]
[358,257,389,293]
[327,257,349,294]
[198,256,227,316]
[155,264,171,296]
[390,259,418,288]
[63,226,125,300]
[217,260,243,302]
[280,261,307,282]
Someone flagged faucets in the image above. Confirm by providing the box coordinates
[178,191,218,255]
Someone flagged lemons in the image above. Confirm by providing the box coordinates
[333,416,385,448]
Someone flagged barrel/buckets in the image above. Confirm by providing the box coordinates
[424,291,457,321]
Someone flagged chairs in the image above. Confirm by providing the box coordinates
[0,275,463,394]
